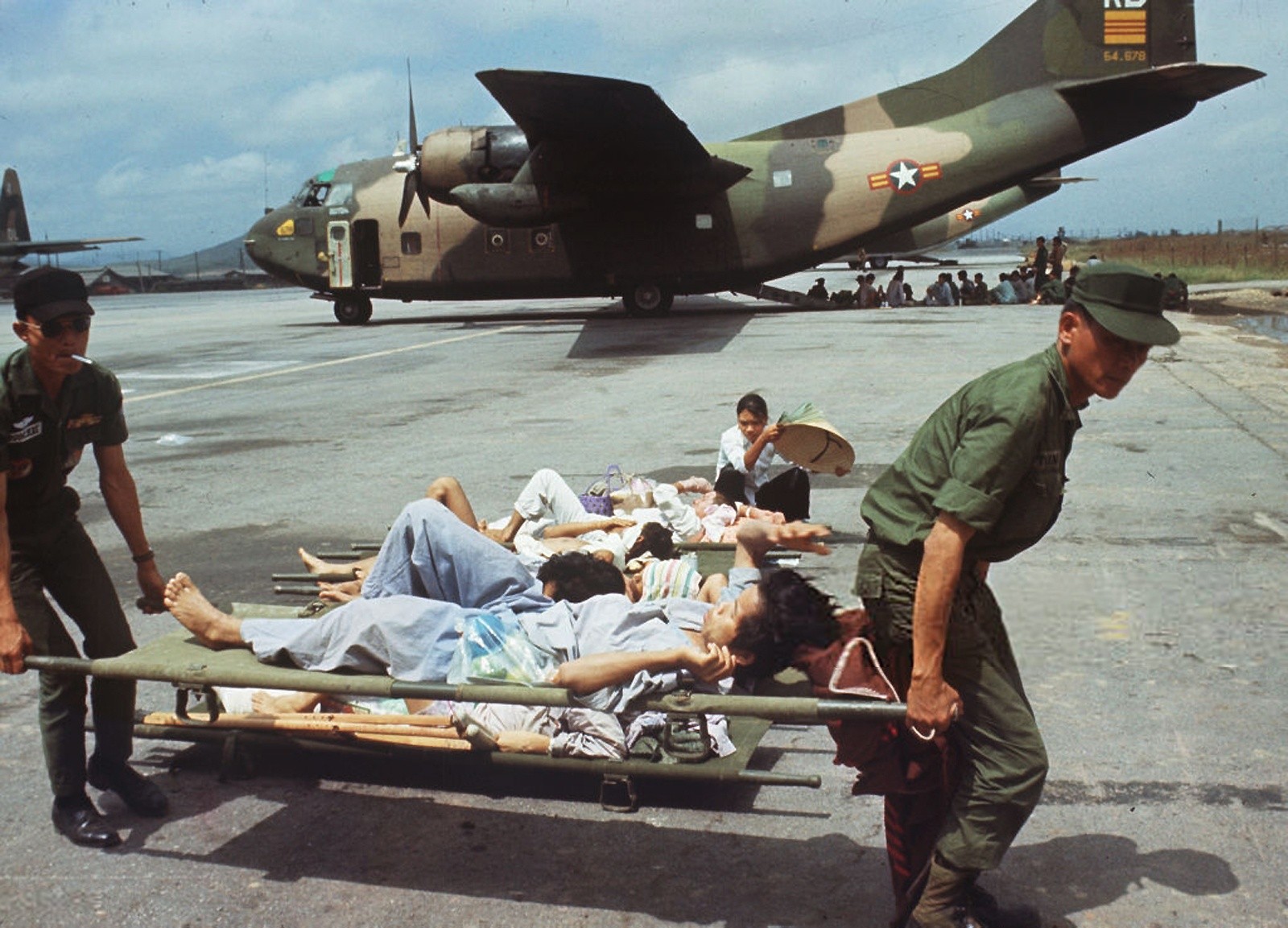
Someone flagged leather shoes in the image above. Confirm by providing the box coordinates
[87,755,168,818]
[52,794,121,847]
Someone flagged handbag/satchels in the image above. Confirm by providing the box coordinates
[579,475,616,518]
[610,472,658,513]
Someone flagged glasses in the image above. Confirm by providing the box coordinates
[17,316,90,338]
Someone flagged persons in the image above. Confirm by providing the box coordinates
[857,263,1182,928]
[805,237,1188,311]
[165,396,838,724]
[0,266,171,850]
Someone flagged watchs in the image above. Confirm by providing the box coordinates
[132,547,155,563]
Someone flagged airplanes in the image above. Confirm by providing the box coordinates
[239,2,1269,319]
[0,167,146,300]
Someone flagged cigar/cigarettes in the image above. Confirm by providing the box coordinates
[72,355,93,364]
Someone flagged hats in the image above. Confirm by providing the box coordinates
[1070,262,1181,346]
[773,408,855,473]
[12,265,95,321]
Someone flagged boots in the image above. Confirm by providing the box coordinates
[904,849,982,928]
